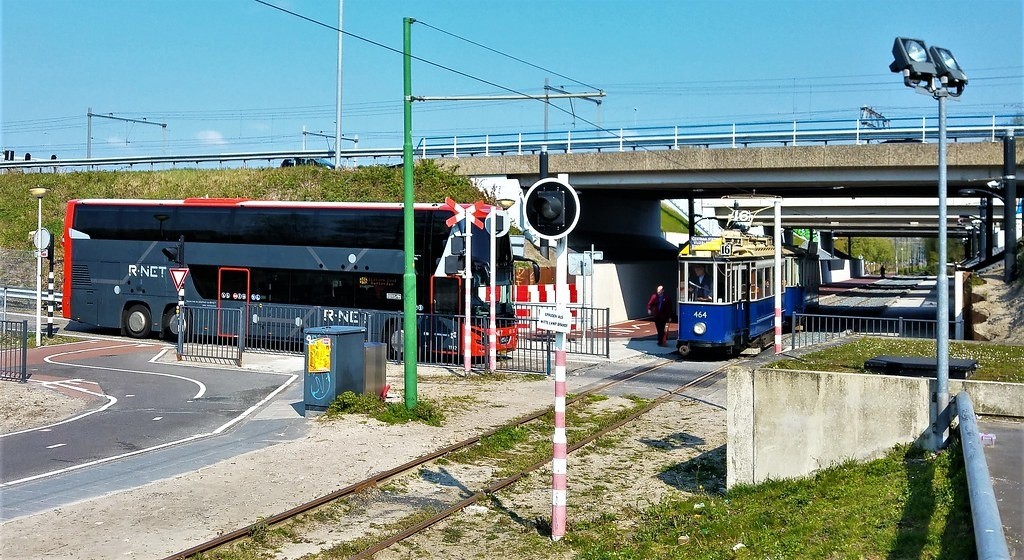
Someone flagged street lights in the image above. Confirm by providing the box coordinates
[28,187,51,343]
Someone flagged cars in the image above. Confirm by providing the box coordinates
[280,157,335,169]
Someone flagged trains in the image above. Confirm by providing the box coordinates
[675,225,822,358]
[889,36,967,450]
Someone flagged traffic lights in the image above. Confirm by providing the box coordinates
[534,189,565,231]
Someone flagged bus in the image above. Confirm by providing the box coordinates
[59,196,518,361]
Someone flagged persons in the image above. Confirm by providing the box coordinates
[880,266,885,277]
[471,287,489,316]
[693,264,713,302]
[647,284,672,346]
[765,279,787,297]
